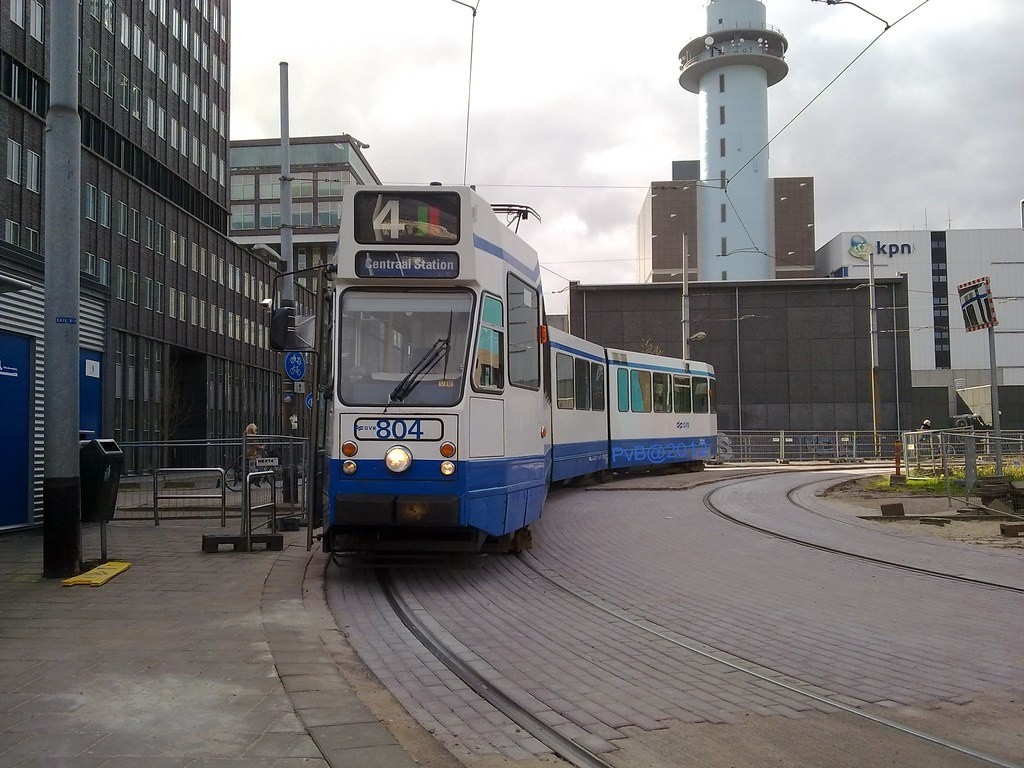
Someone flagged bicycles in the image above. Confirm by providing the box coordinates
[224,458,302,489]
[906,435,956,460]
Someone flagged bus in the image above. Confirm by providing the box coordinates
[267,185,718,559]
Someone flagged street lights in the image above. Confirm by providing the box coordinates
[683,331,706,360]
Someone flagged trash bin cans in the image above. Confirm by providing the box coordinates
[78,430,124,522]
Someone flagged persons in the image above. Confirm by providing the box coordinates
[241,423,268,488]
[918,420,931,454]
